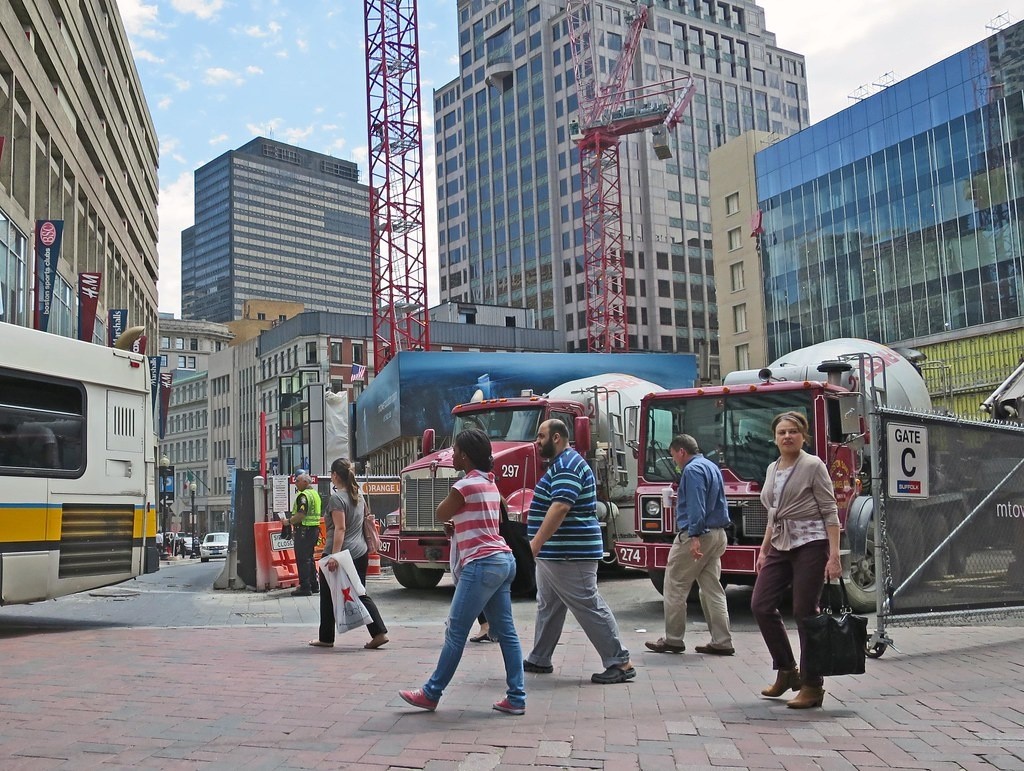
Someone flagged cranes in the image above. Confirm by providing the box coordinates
[364,1,432,374]
[563,0,697,359]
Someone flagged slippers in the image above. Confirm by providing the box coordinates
[590,665,638,683]
[521,659,554,674]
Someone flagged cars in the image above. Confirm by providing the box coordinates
[164,531,199,555]
[198,532,229,562]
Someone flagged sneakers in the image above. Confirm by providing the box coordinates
[491,696,527,716]
[398,689,439,711]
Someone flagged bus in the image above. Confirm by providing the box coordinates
[0,321,160,607]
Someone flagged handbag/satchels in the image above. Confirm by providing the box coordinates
[798,571,869,676]
[364,504,384,556]
[496,495,536,577]
[318,548,373,633]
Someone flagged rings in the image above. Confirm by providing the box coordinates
[695,558,697,562]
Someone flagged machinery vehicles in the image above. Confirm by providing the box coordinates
[613,336,1024,614]
[376,372,673,590]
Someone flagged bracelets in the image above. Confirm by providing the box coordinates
[289,518,292,524]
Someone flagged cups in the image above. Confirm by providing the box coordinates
[661,487,673,508]
[278,512,286,521]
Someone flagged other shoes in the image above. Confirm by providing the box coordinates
[307,639,334,647]
[644,637,687,654]
[469,633,490,643]
[695,644,736,654]
[291,590,312,596]
[311,587,320,594]
[364,633,391,650]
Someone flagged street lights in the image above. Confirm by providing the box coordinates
[189,483,197,559]
[159,457,171,559]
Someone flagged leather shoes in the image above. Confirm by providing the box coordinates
[761,666,801,698]
[786,685,826,710]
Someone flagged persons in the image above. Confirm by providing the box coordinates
[645,435,736,654]
[309,458,389,648]
[470,497,509,642]
[523,419,636,682]
[280,475,321,596]
[399,429,528,713]
[156,531,162,553]
[751,411,843,707]
